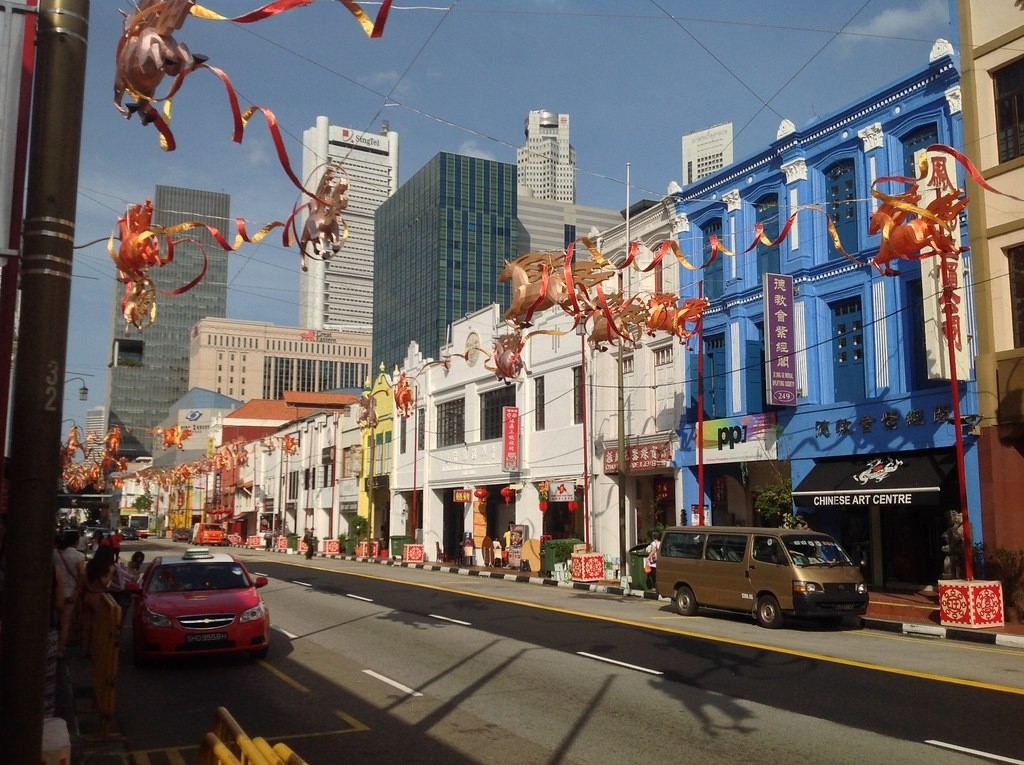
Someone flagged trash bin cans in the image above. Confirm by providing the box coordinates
[345,538,356,556]
[287,536,300,549]
[542,537,587,577]
[628,541,656,591]
[389,535,413,560]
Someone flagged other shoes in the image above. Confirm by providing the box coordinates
[651,587,655,592]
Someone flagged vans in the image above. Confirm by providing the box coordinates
[654,526,870,629]
[192,524,224,545]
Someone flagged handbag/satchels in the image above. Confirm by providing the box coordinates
[643,567,651,574]
[647,541,657,564]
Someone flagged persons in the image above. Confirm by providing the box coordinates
[263,531,273,550]
[646,532,661,589]
[303,527,317,559]
[51,525,144,660]
[503,521,515,547]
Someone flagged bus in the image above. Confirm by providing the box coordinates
[128,515,151,536]
[117,508,139,530]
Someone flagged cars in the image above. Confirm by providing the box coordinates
[85,527,111,542]
[120,529,138,540]
[172,527,193,543]
[125,549,270,666]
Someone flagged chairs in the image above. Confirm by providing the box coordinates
[436,542,447,563]
[464,545,476,568]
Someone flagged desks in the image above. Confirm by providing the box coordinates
[481,537,494,568]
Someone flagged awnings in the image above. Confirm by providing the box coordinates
[792,449,958,508]
[222,512,249,522]
[59,492,114,509]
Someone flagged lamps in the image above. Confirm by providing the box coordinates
[947,415,982,436]
